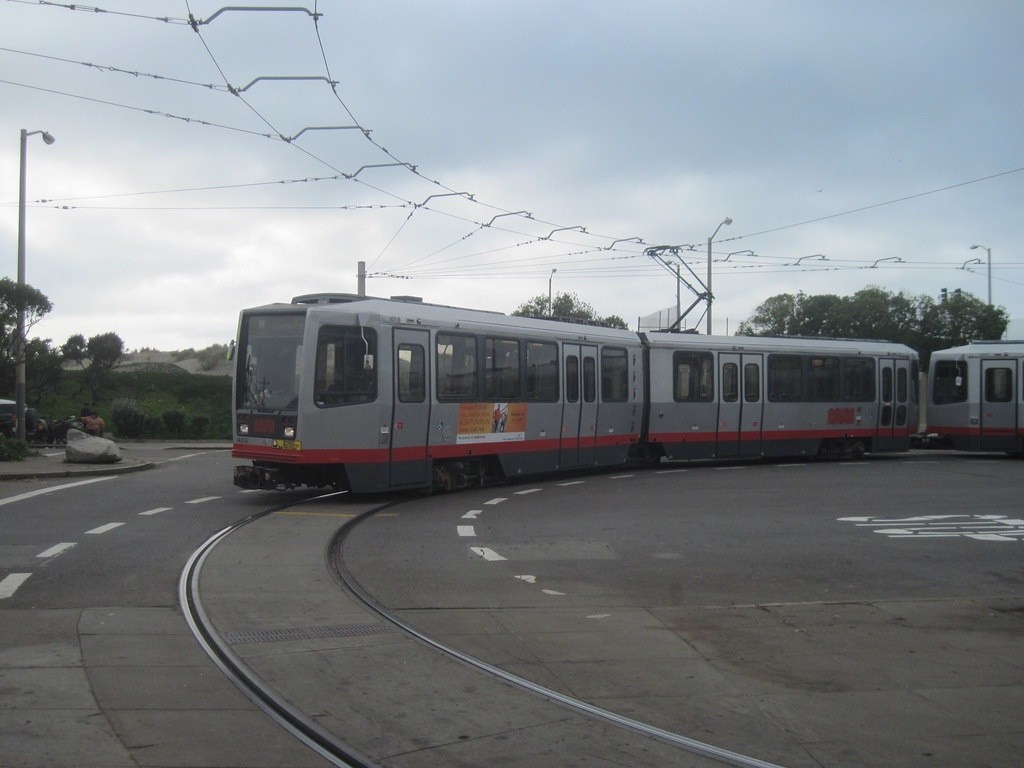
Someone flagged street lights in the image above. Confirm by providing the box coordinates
[707,216,734,334]
[16,126,57,451]
[971,244,993,308]
[548,268,557,318]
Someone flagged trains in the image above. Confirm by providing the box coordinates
[226,262,1024,501]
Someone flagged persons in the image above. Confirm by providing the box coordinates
[76,402,106,436]
